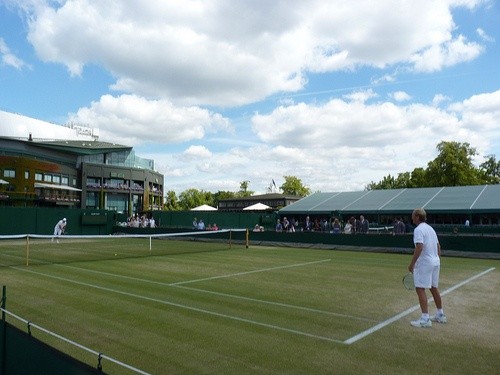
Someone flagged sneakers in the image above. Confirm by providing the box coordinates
[431,312,449,323]
[409,317,432,327]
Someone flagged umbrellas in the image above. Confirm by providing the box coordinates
[243,203,277,226]
[190,204,218,224]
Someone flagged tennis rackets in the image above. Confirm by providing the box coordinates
[403,272,416,290]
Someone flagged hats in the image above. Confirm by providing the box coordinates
[63,217,66,221]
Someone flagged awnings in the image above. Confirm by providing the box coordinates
[0,179,9,185]
[34,183,83,192]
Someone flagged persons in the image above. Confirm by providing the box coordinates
[206,223,213,231]
[212,224,218,231]
[51,217,67,245]
[408,207,447,329]
[192,217,198,230]
[275,218,283,232]
[464,217,470,226]
[282,216,289,232]
[116,213,158,228]
[198,219,205,231]
[289,216,410,234]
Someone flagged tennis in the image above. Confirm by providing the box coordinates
[115,253,117,257]
